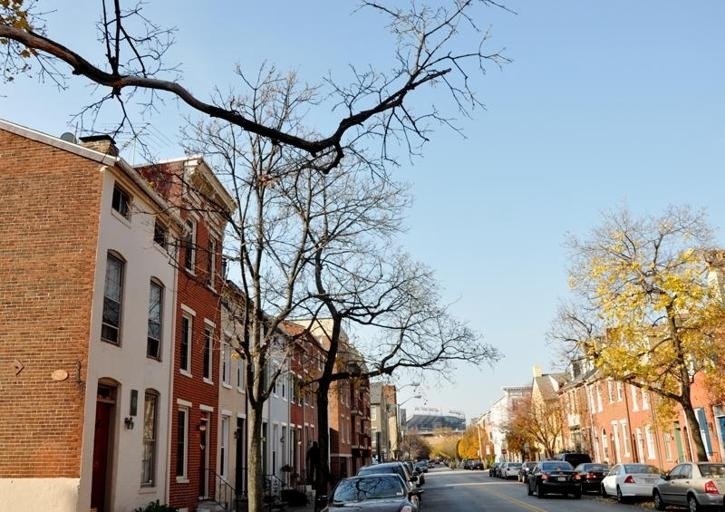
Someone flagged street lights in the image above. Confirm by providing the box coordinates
[397,395,422,451]
[385,382,420,460]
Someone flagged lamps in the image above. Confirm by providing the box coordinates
[125,417,134,429]
[234,429,239,439]
[198,420,207,431]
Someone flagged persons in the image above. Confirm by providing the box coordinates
[452,457,457,469]
[498,454,504,463]
[306,441,319,482]
[450,459,454,471]
[482,456,487,471]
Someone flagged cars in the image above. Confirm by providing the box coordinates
[320,457,435,512]
[489,450,725,512]
[443,457,486,471]
